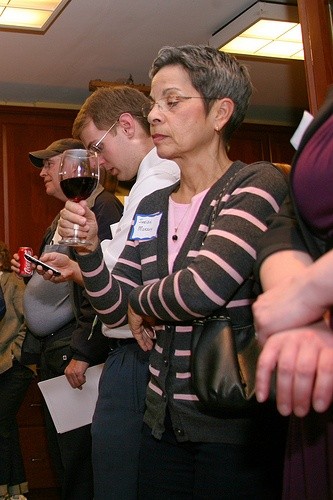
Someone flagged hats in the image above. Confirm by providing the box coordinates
[29,138,85,169]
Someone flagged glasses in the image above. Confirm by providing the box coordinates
[142,94,223,117]
[86,114,140,159]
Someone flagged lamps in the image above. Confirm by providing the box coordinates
[208,0,305,62]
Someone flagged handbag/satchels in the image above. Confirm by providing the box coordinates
[190,313,277,419]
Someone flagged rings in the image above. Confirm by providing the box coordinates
[254,330,259,341]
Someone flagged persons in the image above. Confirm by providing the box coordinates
[251,92,333,500]
[57,44,289,500]
[0,239,29,500]
[32,86,182,500]
[9,137,125,500]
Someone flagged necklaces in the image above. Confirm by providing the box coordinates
[170,163,224,241]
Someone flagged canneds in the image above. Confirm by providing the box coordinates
[17,247,33,276]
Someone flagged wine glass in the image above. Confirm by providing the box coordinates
[57,148,100,247]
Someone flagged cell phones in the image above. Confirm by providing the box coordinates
[25,254,62,276]
